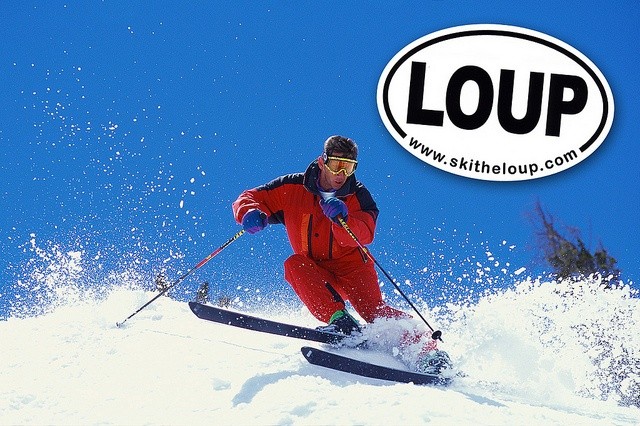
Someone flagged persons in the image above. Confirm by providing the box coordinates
[230,135,453,375]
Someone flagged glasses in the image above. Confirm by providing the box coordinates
[322,152,358,177]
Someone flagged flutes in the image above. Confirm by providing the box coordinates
[189,302,453,387]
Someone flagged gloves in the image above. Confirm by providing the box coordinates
[241,208,268,234]
[319,197,348,226]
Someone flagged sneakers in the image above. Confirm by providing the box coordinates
[315,313,366,336]
[423,350,469,377]
